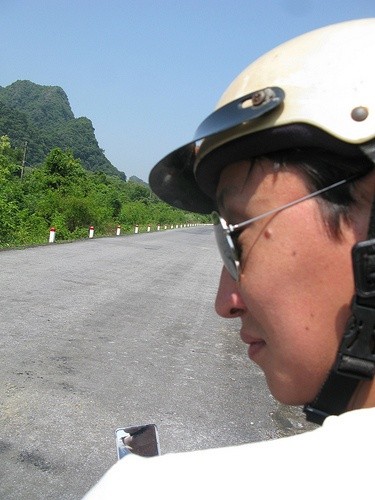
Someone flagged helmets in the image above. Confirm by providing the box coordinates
[148,18,375,215]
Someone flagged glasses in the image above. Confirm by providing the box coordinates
[209,172,370,282]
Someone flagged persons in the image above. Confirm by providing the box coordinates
[82,19,375,500]
[117,424,158,460]
